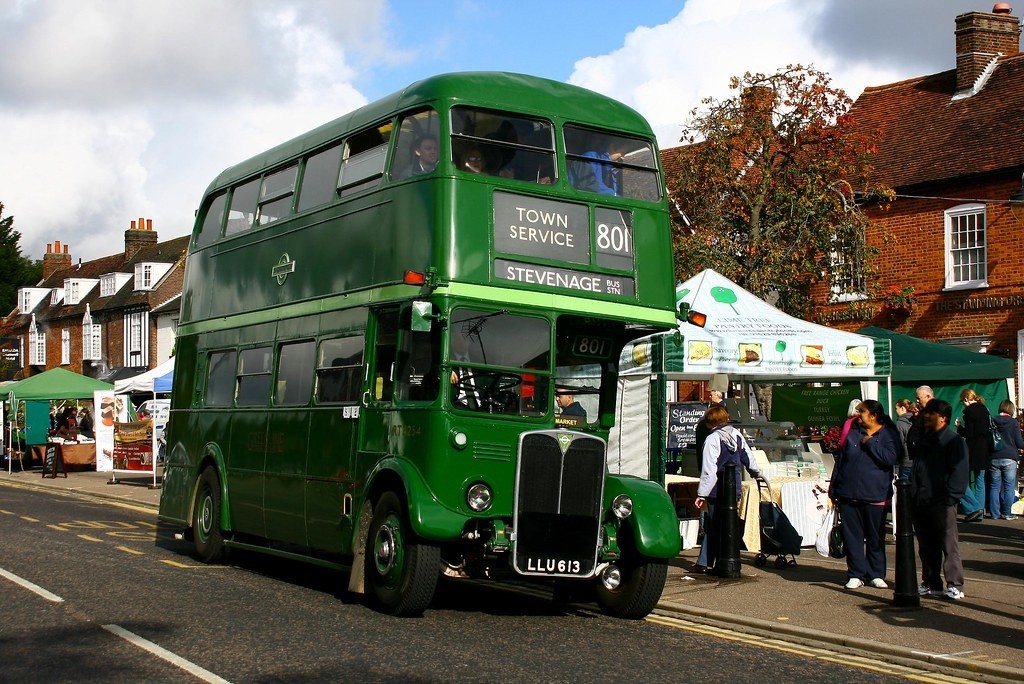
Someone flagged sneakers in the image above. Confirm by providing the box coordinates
[845,578,865,589]
[918,586,944,595]
[870,577,888,588]
[943,587,964,600]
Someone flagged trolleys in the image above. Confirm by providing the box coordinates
[755,475,803,569]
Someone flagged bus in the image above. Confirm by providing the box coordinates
[158,71,707,619]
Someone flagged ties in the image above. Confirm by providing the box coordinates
[459,357,478,410]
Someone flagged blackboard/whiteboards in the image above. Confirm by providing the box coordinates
[43,443,67,473]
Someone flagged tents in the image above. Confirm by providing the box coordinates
[852,326,1016,496]
[113,356,175,487]
[0,368,115,475]
[553,269,898,542]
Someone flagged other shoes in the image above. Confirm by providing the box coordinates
[965,509,983,522]
[1006,516,1019,521]
[687,564,707,574]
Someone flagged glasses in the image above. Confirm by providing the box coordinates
[959,399,963,403]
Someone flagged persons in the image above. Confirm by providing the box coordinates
[399,136,438,178]
[159,422,169,457]
[141,410,150,420]
[556,388,587,419]
[462,136,630,199]
[896,399,919,477]
[837,398,862,449]
[829,400,905,589]
[710,390,726,406]
[990,399,1024,520]
[50,405,94,438]
[955,388,992,523]
[908,399,970,599]
[424,330,513,412]
[913,386,934,417]
[689,405,760,573]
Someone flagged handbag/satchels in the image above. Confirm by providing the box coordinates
[828,504,846,559]
[988,415,1003,452]
[815,509,834,558]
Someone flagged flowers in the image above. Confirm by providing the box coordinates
[882,282,918,322]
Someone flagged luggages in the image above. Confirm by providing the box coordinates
[751,472,803,569]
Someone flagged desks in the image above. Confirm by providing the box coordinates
[33,442,96,471]
[665,474,701,517]
[737,476,830,553]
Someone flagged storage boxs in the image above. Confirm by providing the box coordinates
[758,455,827,479]
